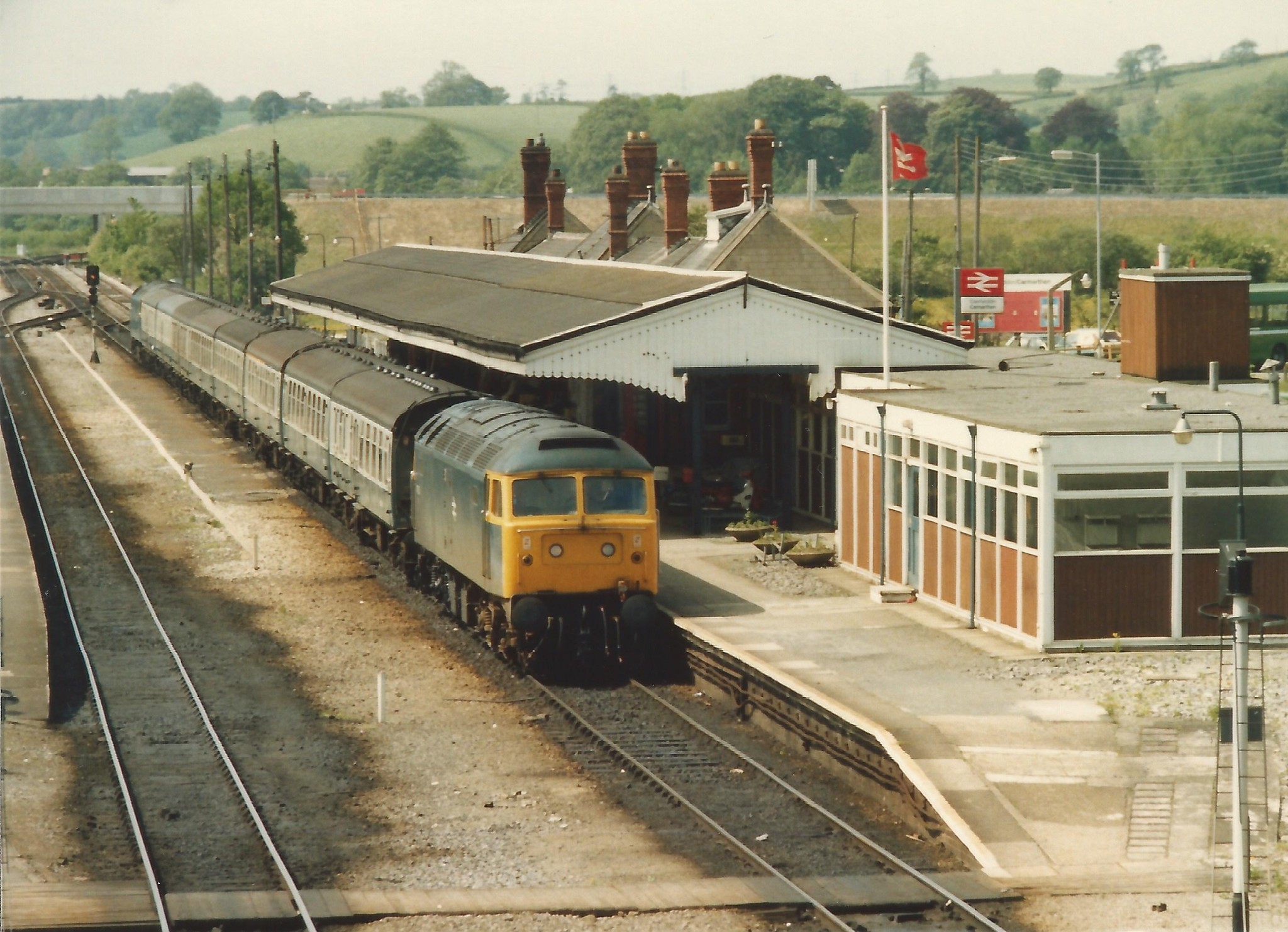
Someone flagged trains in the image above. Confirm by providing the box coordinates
[128,280,660,671]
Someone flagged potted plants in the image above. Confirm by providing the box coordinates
[725,520,835,566]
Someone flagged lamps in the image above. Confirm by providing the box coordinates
[826,397,838,410]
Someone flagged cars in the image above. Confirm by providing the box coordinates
[1003,326,1122,363]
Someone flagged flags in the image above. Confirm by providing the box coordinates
[889,132,928,181]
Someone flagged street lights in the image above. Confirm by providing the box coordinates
[302,233,327,337]
[331,237,358,346]
[1047,268,1093,351]
[889,185,931,322]
[973,153,1020,345]
[1170,407,1254,932]
[1050,149,1102,360]
[248,230,268,316]
[275,231,297,325]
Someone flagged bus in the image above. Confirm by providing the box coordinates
[1249,280,1288,371]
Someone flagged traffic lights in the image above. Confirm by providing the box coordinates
[86,265,100,286]
